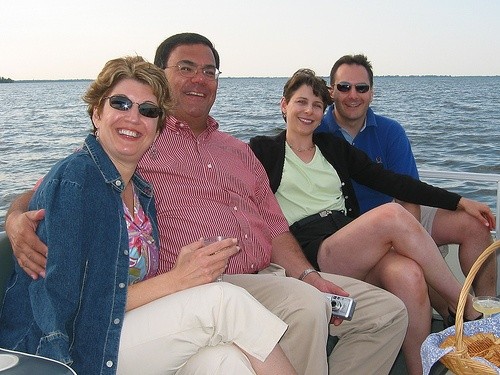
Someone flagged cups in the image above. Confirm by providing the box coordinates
[201,236,229,282]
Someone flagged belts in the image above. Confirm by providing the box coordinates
[292,210,339,233]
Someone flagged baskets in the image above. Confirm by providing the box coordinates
[438,240,500,375]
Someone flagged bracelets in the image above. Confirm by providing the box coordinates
[299,269,321,281]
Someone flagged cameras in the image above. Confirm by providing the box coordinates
[330,295,356,320]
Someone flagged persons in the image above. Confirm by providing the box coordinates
[4,33,408,375]
[313,55,500,318]
[0,56,296,375]
[248,68,497,375]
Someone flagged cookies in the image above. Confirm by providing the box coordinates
[439,332,500,371]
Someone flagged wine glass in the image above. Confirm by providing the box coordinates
[472,296,500,318]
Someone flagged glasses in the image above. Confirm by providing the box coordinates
[332,81,371,93]
[163,63,221,80]
[102,94,163,119]
